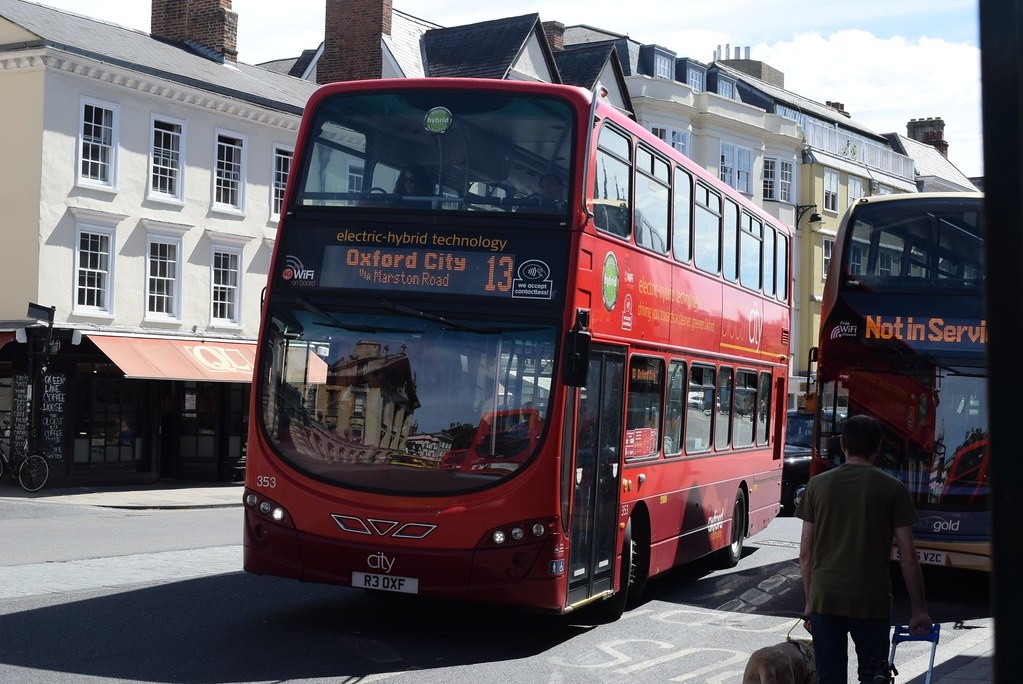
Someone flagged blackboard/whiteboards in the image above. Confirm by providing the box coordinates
[39,372,70,469]
[9,374,34,463]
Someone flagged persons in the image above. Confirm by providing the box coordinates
[795,416,932,684]
[392,165,434,209]
[540,174,563,199]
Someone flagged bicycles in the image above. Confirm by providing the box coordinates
[0,420,51,493]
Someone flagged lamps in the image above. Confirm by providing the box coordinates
[49,340,62,356]
[796,203,826,230]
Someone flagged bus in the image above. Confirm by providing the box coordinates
[803,193,991,570]
[244,76,796,623]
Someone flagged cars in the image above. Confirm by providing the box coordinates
[780,406,848,518]
[687,381,770,424]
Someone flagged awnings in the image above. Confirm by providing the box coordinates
[87,334,328,384]
[504,371,586,399]
[499,382,512,397]
[0,331,18,348]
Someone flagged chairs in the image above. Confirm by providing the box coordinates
[846,274,981,292]
[356,188,391,206]
